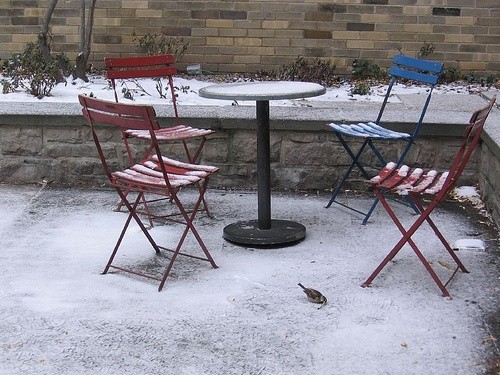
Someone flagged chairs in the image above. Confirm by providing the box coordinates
[326,54,444,224]
[77,94,216,291]
[104,54,216,224]
[361,97,496,293]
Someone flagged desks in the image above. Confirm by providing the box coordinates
[198,81,325,248]
